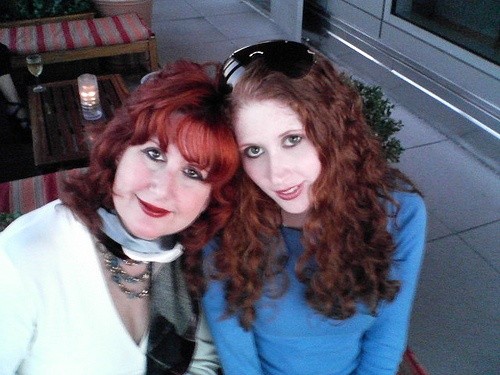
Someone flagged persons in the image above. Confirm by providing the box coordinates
[0,43,31,130]
[0,56,237,375]
[199,40,427,375]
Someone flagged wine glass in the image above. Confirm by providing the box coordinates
[26,54,45,92]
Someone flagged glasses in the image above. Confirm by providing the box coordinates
[216,40,334,98]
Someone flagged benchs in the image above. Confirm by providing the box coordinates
[1,12,159,76]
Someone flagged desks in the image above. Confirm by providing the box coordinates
[27,74,133,171]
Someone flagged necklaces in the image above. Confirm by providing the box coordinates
[96,241,153,300]
